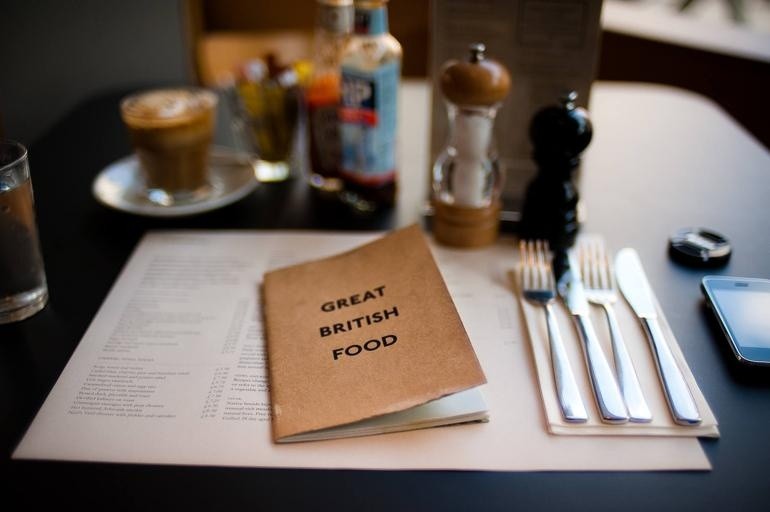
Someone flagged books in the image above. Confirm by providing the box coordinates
[262,221,492,445]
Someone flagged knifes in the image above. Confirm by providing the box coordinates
[617,245,703,425]
[555,247,628,426]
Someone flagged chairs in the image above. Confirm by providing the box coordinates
[2,2,211,94]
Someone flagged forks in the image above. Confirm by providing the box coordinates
[520,235,589,422]
[578,242,651,422]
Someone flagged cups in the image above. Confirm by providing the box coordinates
[233,58,340,195]
[0,141,48,326]
[123,86,218,200]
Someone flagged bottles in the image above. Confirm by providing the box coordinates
[516,92,592,245]
[427,45,512,241]
[337,2,402,185]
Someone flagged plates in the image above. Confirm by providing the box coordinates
[95,151,262,219]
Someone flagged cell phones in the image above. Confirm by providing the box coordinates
[699,276,770,366]
